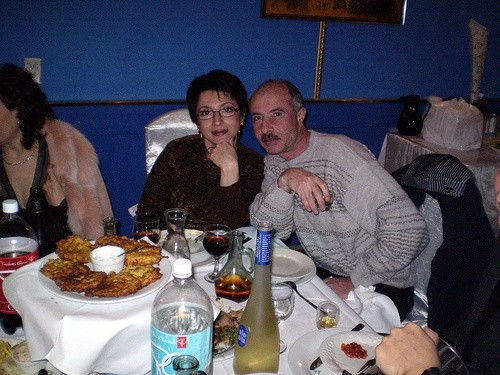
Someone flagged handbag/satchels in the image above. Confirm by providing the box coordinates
[422,98,483,151]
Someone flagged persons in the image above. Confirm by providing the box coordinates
[375,224,500,375]
[134,69,265,235]
[249,78,429,323]
[0,62,117,259]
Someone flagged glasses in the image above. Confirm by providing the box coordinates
[196,107,240,120]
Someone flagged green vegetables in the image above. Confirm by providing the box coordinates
[210,325,238,355]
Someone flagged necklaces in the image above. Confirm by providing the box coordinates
[3,140,39,165]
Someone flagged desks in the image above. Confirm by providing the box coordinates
[377,132,500,240]
[0,226,376,375]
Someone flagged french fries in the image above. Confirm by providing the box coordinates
[0,340,19,375]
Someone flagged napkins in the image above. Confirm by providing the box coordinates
[329,276,401,334]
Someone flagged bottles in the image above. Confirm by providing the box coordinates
[0,199,39,336]
[163,208,190,260]
[421,95,442,119]
[233,222,280,375]
[149,258,214,375]
[473,92,497,137]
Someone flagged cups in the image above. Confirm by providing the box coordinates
[11,341,46,375]
[133,213,160,246]
[90,246,125,275]
[315,301,340,330]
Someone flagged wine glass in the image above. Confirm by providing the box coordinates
[271,282,295,353]
[202,225,232,283]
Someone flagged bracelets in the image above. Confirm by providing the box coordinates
[420,367,441,375]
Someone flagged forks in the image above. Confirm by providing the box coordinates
[284,281,328,315]
[356,358,376,374]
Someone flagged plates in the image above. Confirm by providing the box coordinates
[160,229,226,269]
[210,296,245,361]
[271,248,317,286]
[38,250,171,304]
[319,331,383,375]
[288,328,353,375]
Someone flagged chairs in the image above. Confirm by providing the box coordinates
[144,108,199,174]
[391,152,483,328]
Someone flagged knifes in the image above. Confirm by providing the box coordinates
[309,323,365,370]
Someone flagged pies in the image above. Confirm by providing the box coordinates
[40,235,163,297]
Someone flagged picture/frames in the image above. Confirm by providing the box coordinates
[260,0,407,25]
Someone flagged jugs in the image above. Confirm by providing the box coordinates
[214,230,255,304]
[397,94,432,137]
